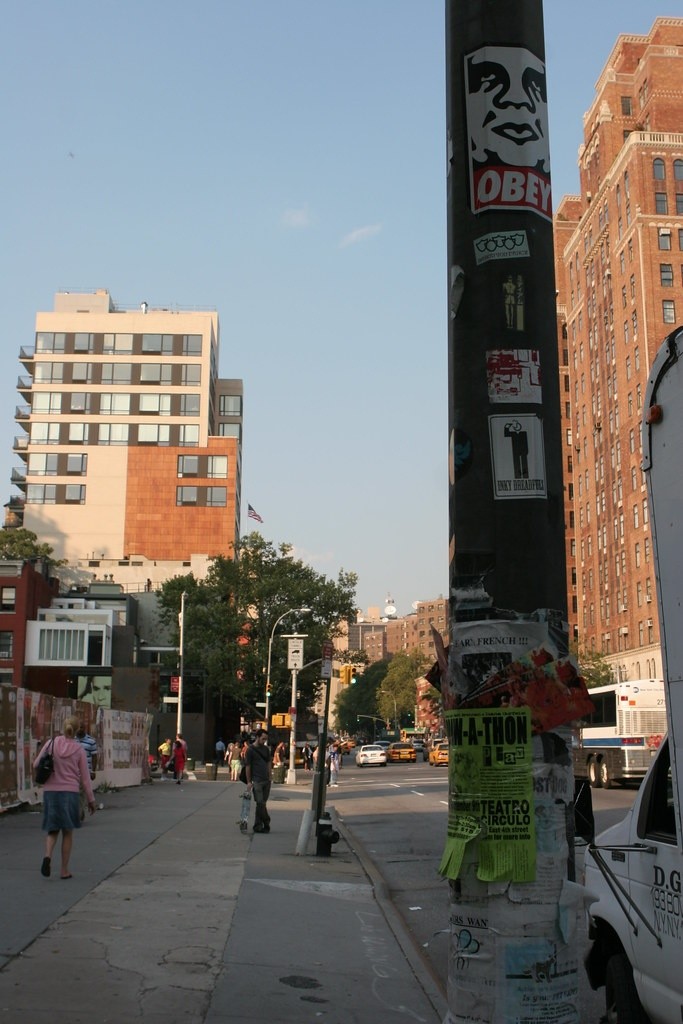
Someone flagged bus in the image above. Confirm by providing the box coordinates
[577,678,668,789]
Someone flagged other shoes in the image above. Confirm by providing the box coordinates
[254,827,264,833]
[263,816,270,833]
[331,784,338,787]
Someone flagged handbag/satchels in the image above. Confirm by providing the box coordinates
[35,736,56,784]
[239,767,252,784]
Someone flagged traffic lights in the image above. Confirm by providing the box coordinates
[349,666,357,684]
[340,665,348,684]
[267,684,271,697]
[271,685,274,696]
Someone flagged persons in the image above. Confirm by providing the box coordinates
[324,738,344,788]
[274,741,289,766]
[302,742,319,771]
[166,741,187,785]
[244,729,273,833]
[214,738,250,784]
[158,738,172,781]
[172,733,188,751]
[33,714,98,879]
[73,722,98,820]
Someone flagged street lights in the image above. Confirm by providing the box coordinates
[172,590,189,740]
[263,608,314,744]
[382,690,397,741]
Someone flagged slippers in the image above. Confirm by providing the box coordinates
[41,857,50,876]
[61,874,71,879]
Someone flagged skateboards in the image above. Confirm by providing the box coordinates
[236,788,252,835]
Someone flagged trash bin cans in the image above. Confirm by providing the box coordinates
[186,758,195,771]
[205,761,218,780]
[272,765,286,784]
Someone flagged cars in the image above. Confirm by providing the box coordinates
[332,735,392,756]
[355,744,387,768]
[386,741,417,763]
[410,736,450,767]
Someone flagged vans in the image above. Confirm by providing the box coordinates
[571,730,683,1024]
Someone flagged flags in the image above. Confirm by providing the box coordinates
[248,504,264,523]
